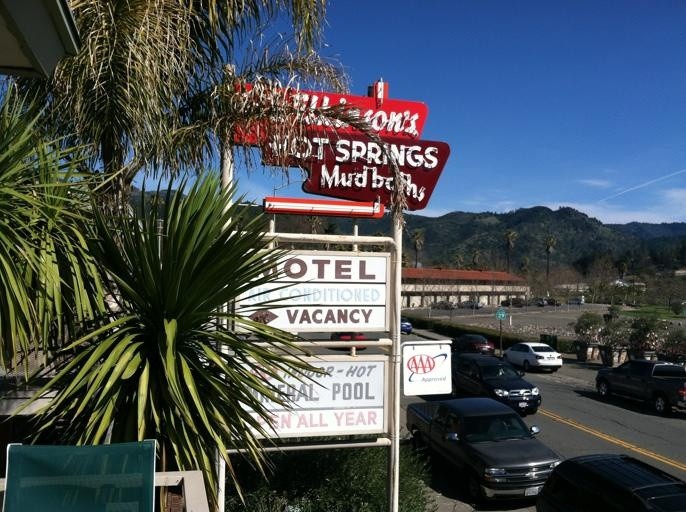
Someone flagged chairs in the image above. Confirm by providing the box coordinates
[2,439,160,512]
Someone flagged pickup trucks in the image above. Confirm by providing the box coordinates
[406,398,566,500]
[596,360,686,414]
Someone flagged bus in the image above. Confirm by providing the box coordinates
[566,296,584,305]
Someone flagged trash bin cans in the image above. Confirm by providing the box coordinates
[540,335,557,350]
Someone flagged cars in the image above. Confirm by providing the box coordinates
[431,301,458,310]
[450,334,495,354]
[501,298,561,308]
[537,455,686,512]
[401,318,413,334]
[331,332,366,350]
[503,342,563,372]
[451,353,541,412]
[462,300,483,310]
